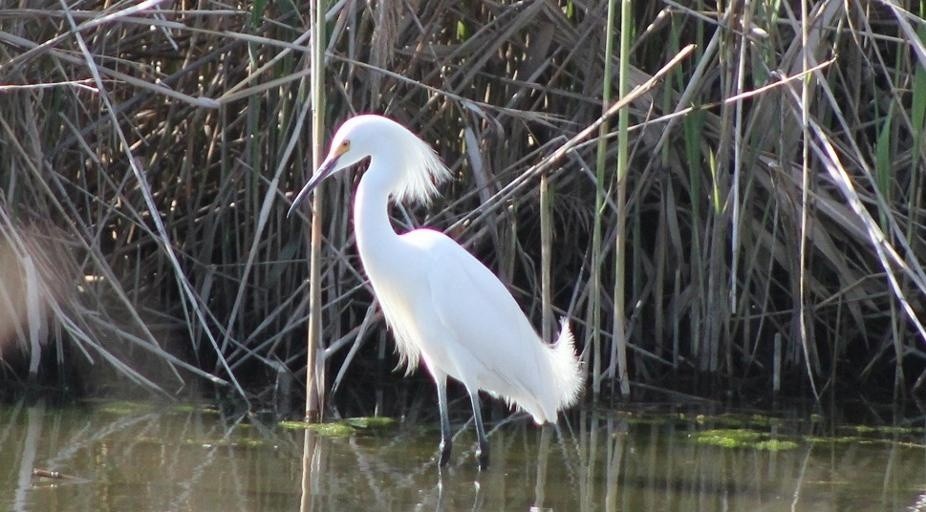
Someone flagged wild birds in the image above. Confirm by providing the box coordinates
[284,112,587,475]
